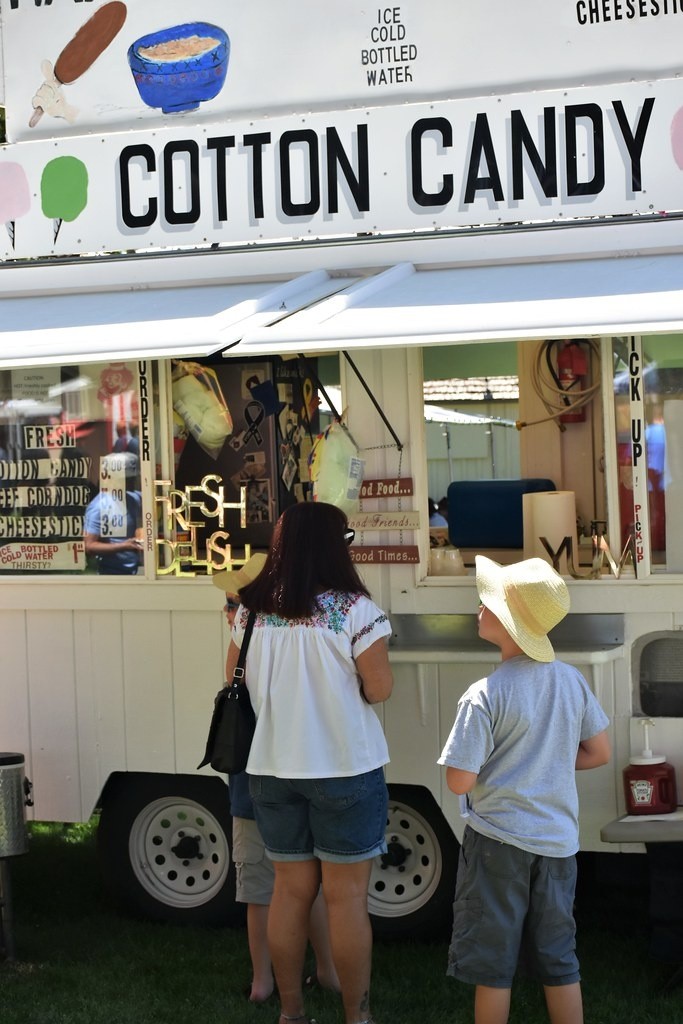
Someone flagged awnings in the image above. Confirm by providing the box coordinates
[0,254,683,370]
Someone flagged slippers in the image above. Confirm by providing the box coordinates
[307,972,348,993]
[243,972,277,1001]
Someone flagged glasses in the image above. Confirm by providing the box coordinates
[344,528,355,545]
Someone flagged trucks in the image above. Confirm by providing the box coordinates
[0,216,682,937]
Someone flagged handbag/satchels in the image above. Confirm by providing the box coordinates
[198,611,258,775]
[172,358,233,459]
[308,418,365,516]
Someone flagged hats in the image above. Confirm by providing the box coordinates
[103,452,138,477]
[474,553,571,663]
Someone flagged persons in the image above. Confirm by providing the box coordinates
[428,497,449,527]
[619,394,673,493]
[109,420,140,454]
[436,555,612,1024]
[212,501,394,1024]
[82,453,144,576]
